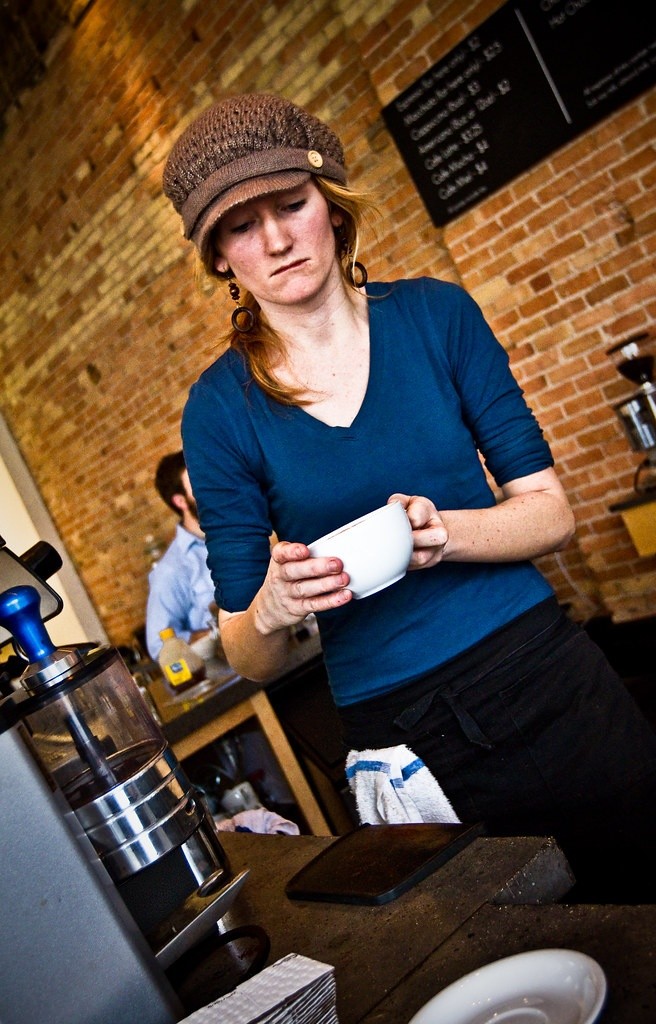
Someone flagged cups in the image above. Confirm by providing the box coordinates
[301,502,418,600]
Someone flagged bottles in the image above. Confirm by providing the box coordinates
[157,628,205,693]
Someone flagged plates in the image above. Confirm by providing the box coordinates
[407,948,606,1024]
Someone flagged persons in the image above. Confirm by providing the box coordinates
[160,95,653,900]
[145,451,230,664]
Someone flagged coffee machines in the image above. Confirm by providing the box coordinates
[606,324,656,500]
[0,537,268,1023]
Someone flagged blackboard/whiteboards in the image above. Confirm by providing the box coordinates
[380,0,656,230]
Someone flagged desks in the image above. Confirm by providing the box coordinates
[32,622,331,838]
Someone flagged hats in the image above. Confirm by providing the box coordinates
[162,93,348,280]
